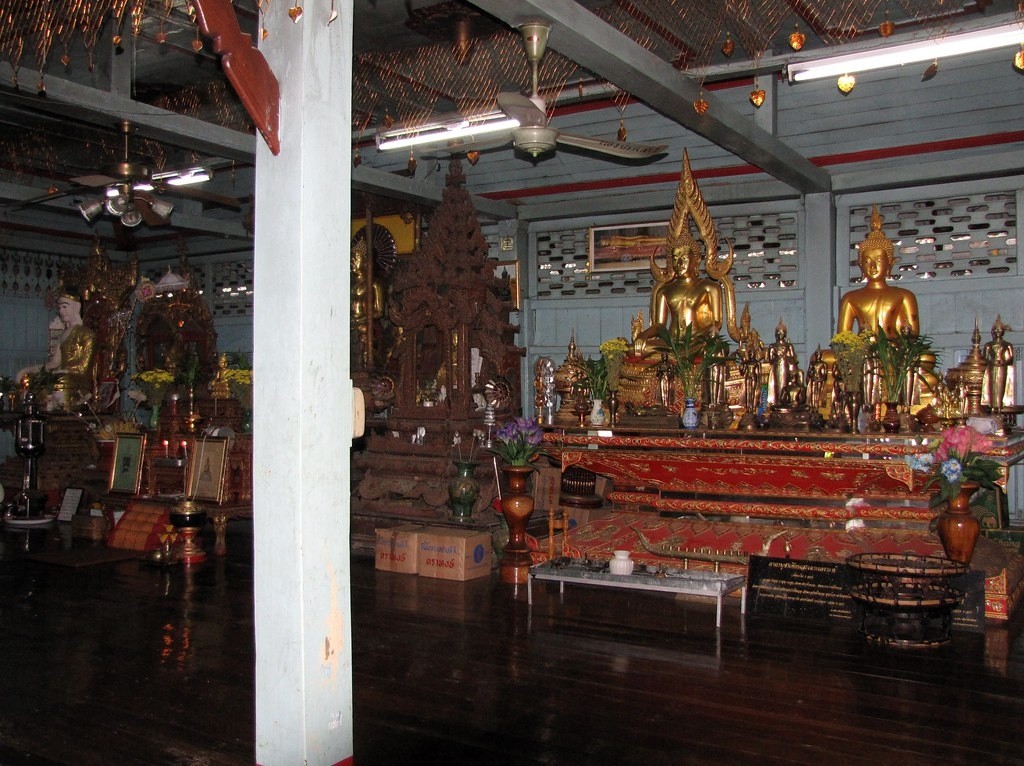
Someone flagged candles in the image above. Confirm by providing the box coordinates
[165,440,168,456]
[183,441,188,456]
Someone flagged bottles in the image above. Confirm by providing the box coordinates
[609,550,634,576]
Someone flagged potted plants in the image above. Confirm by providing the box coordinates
[866,325,951,433]
[571,357,605,427]
[34,362,66,413]
[643,321,741,430]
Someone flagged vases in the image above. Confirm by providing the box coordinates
[846,391,860,436]
[149,406,163,430]
[450,459,483,523]
[242,409,252,431]
[608,389,619,427]
[498,466,536,583]
[937,484,980,567]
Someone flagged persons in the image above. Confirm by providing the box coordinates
[808,344,828,408]
[983,313,1014,411]
[740,349,761,412]
[627,231,723,362]
[811,203,936,373]
[351,238,385,331]
[15,285,95,400]
[439,329,457,374]
[211,351,231,387]
[768,319,794,401]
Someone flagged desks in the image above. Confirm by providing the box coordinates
[526,557,747,628]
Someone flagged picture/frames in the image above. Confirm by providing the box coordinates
[588,220,689,274]
[187,435,230,501]
[106,432,148,495]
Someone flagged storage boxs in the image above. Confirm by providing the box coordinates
[375,524,418,575]
[416,526,494,581]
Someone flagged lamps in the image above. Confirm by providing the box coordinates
[106,172,215,197]
[786,11,1024,85]
[76,184,174,227]
[374,112,521,153]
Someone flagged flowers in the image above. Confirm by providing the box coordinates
[223,369,251,409]
[130,368,175,405]
[829,326,876,391]
[920,420,1003,509]
[601,337,630,390]
[484,417,562,476]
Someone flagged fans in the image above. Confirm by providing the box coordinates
[70,123,241,228]
[421,23,669,163]
